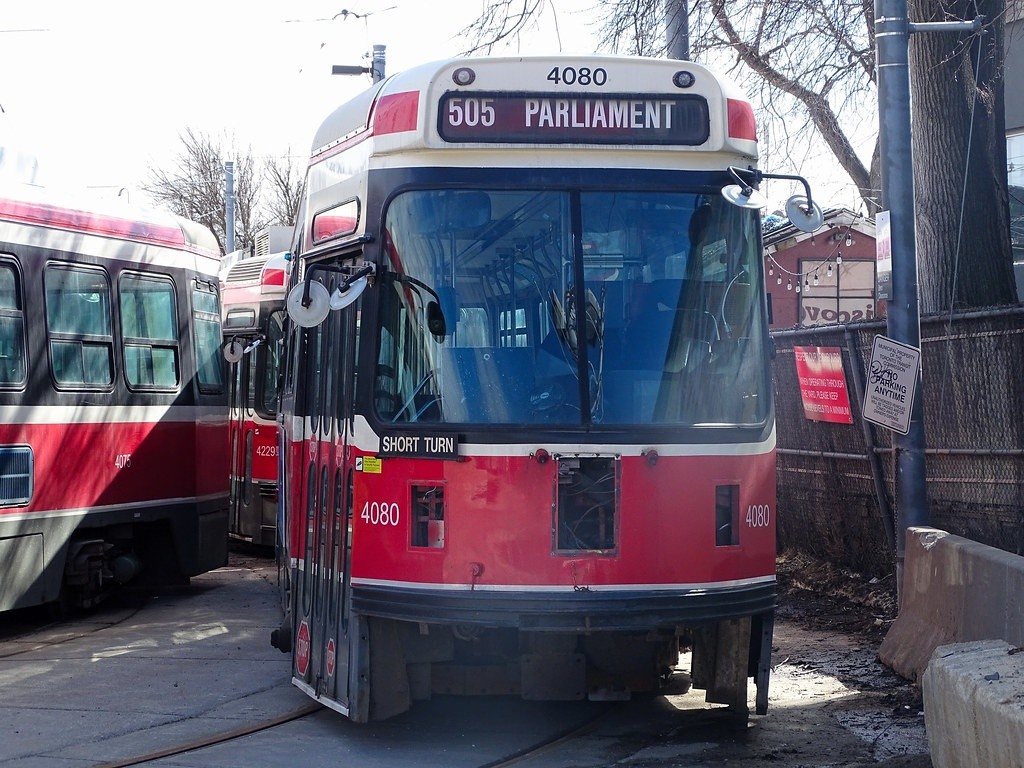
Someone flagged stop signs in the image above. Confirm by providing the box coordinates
[295,621,310,676]
[327,638,335,676]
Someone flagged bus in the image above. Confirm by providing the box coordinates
[271,60,822,727]
[0,195,490,615]
